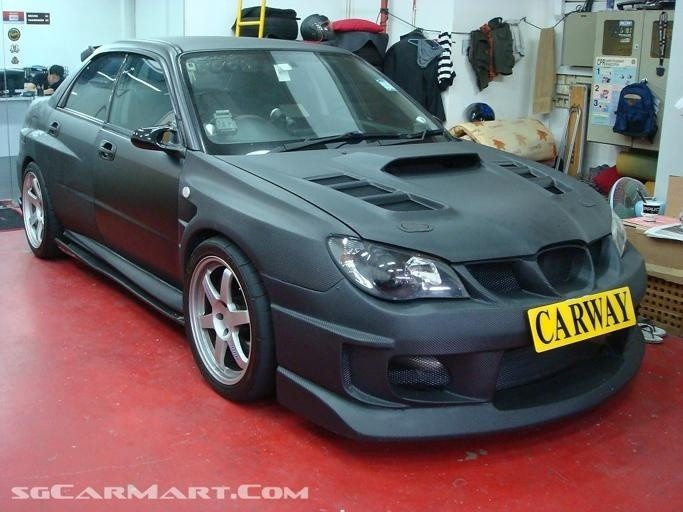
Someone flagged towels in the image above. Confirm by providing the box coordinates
[532,27,557,116]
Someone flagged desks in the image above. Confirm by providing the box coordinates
[0,95,42,159]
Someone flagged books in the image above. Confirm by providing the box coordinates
[623,215,681,230]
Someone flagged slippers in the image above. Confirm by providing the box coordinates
[637,322,667,344]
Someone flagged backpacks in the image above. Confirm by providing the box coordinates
[613,80,658,147]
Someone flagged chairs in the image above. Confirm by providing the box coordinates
[0,70,24,96]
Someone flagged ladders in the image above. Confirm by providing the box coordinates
[235,0,266,38]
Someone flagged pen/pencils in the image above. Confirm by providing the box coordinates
[36,82,45,90]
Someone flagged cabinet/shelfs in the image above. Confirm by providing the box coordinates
[587,9,674,154]
[559,11,602,69]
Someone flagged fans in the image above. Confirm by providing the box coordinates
[608,176,648,221]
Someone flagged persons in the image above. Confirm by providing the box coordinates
[27,64,65,96]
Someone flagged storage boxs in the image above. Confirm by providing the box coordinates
[622,174,682,273]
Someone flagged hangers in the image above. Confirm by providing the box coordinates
[407,29,444,53]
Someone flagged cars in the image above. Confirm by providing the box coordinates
[14,34,653,458]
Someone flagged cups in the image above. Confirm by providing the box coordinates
[642,203,661,222]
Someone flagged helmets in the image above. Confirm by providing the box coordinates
[300,15,335,42]
[465,103,494,121]
